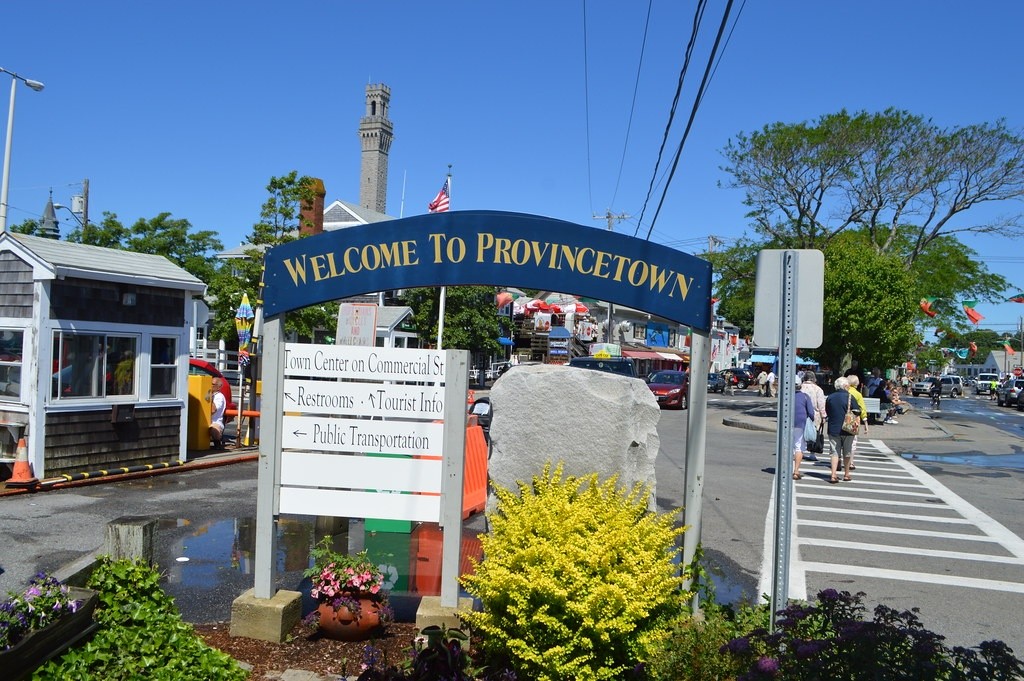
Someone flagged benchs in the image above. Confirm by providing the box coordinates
[851,397,881,415]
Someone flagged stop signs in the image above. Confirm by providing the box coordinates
[1014,369,1022,376]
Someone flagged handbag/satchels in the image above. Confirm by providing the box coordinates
[807,423,824,452]
[841,394,860,435]
[804,417,816,441]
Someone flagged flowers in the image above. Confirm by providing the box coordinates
[297,535,395,635]
[0,564,85,653]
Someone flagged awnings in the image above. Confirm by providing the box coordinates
[749,355,819,365]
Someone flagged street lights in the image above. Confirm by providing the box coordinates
[0,67,45,233]
[1007,337,1024,369]
[53,203,88,243]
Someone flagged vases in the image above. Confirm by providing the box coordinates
[0,584,100,681]
[316,589,386,634]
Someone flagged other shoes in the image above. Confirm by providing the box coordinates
[836,461,841,471]
[891,416,897,418]
[886,418,898,424]
[849,463,855,469]
[902,408,909,415]
[213,440,221,450]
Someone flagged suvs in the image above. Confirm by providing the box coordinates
[975,373,1000,395]
[911,375,962,398]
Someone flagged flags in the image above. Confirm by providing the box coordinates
[428,178,449,213]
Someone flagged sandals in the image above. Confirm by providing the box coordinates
[843,476,851,481]
[830,477,839,482]
[793,473,801,479]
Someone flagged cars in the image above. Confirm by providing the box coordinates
[995,380,1024,408]
[720,368,755,389]
[707,373,725,393]
[648,370,689,409]
[569,349,650,384]
[1016,385,1024,411]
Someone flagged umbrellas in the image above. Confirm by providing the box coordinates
[234,290,255,446]
[523,298,589,318]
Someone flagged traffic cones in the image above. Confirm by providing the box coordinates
[5,438,39,486]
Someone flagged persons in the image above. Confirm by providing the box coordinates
[990,378,997,400]
[868,369,909,424]
[793,370,869,483]
[208,377,226,450]
[930,378,942,396]
[756,368,776,397]
